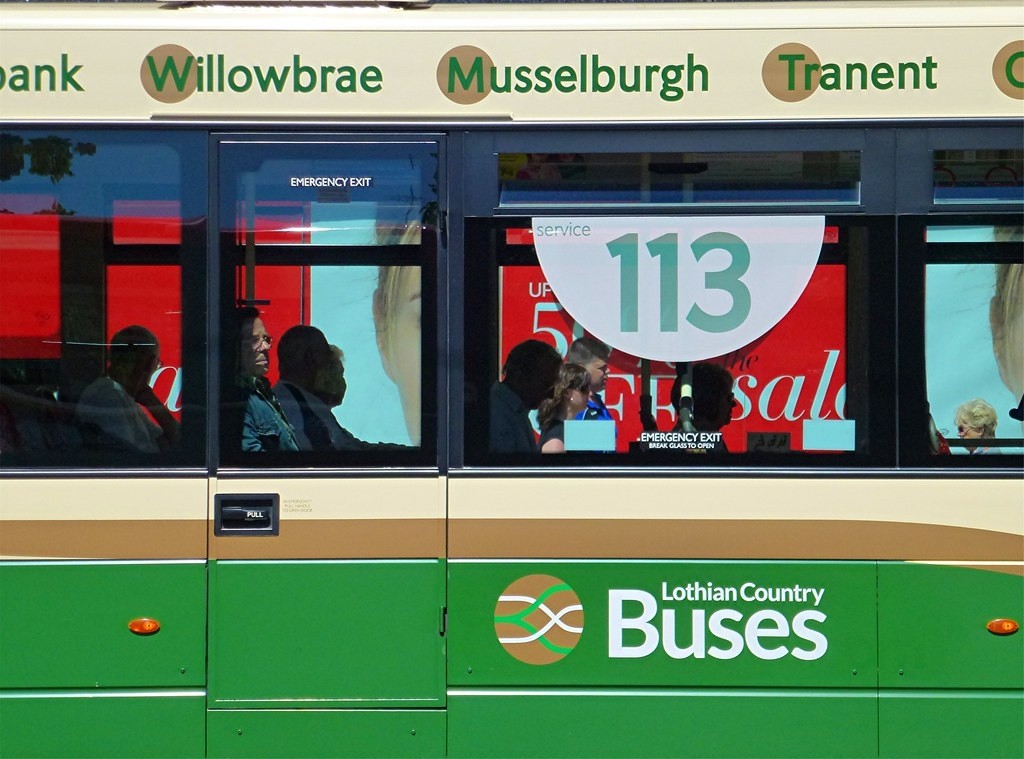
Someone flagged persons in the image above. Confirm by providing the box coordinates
[371,220,426,446]
[668,362,741,455]
[70,322,185,465]
[484,338,567,461]
[564,337,618,457]
[951,397,1010,457]
[227,304,420,452]
[530,365,597,456]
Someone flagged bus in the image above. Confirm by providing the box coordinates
[0,0,1022,759]
